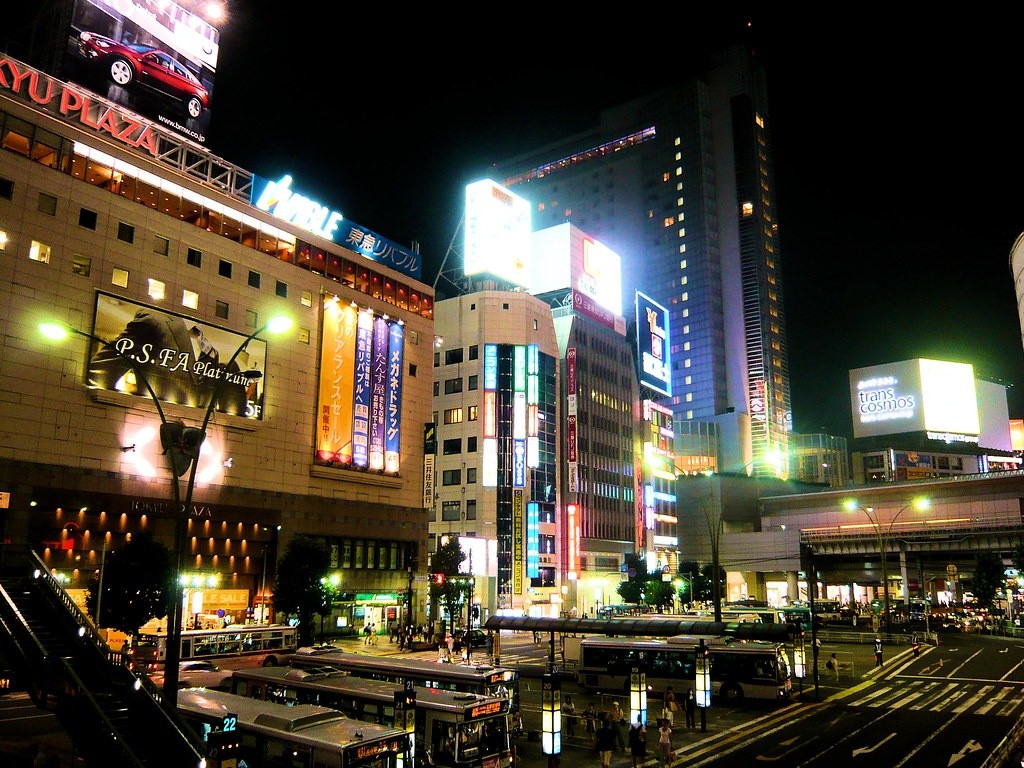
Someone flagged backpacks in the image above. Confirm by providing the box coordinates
[826,659,834,670]
[629,724,643,747]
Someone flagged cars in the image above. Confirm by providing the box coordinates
[452,629,489,649]
[76,31,209,119]
[149,660,237,692]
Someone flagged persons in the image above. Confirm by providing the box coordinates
[121,639,130,668]
[486,630,494,657]
[532,630,542,648]
[830,653,840,686]
[811,635,821,658]
[561,686,697,768]
[188,617,230,630]
[364,622,377,645]
[874,638,883,667]
[912,631,920,657]
[388,622,473,665]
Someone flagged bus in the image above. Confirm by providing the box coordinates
[599,604,640,619]
[231,665,512,768]
[177,684,414,768]
[289,647,521,733]
[692,598,843,625]
[129,622,297,670]
[871,600,931,618]
[580,636,795,706]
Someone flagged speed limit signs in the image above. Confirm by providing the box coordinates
[663,565,671,574]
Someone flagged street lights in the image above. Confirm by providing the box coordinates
[651,451,785,623]
[42,309,298,718]
[843,496,932,642]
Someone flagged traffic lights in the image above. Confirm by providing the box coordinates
[437,575,445,583]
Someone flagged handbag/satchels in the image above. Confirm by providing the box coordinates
[619,708,626,727]
[682,698,687,711]
[668,702,678,712]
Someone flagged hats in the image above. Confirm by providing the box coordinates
[613,702,619,705]
[876,639,881,643]
